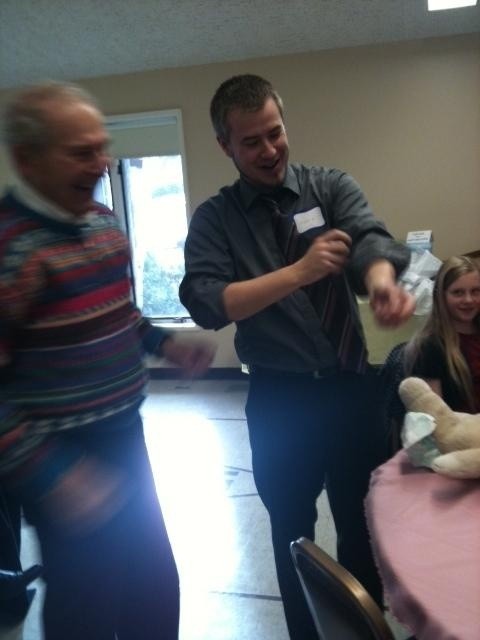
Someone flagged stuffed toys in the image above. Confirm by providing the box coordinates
[399,376,479,479]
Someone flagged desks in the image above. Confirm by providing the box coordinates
[362,447,480,640]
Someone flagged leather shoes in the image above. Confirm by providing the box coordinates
[0,564,43,593]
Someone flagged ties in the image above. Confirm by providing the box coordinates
[259,196,369,375]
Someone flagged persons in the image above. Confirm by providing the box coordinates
[395,254,479,412]
[179,75,418,640]
[1,81,219,639]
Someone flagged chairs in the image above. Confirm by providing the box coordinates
[290,537,395,640]
